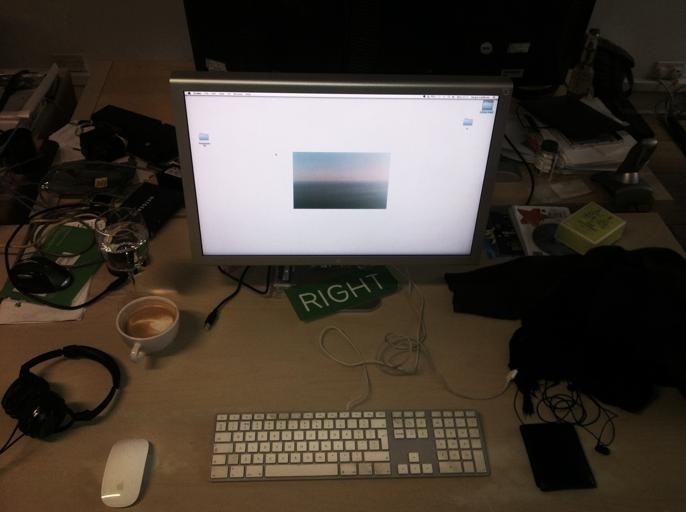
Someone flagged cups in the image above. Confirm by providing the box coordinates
[116,295,180,363]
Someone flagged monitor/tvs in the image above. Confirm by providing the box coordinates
[168,77,514,290]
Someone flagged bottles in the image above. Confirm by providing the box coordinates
[534,140,559,182]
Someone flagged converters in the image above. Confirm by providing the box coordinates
[156,164,183,195]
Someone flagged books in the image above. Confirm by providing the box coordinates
[517,97,638,199]
[509,205,574,256]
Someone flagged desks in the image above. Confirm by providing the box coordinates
[2,0,686,511]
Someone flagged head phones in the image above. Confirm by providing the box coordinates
[1,344,120,440]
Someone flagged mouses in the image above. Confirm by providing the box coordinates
[9,255,72,293]
[100,438,149,508]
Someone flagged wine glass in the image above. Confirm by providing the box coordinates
[94,207,151,308]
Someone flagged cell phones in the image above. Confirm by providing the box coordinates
[519,422,597,491]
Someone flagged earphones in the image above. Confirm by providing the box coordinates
[595,445,609,455]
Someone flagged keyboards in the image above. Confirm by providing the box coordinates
[208,408,491,483]
